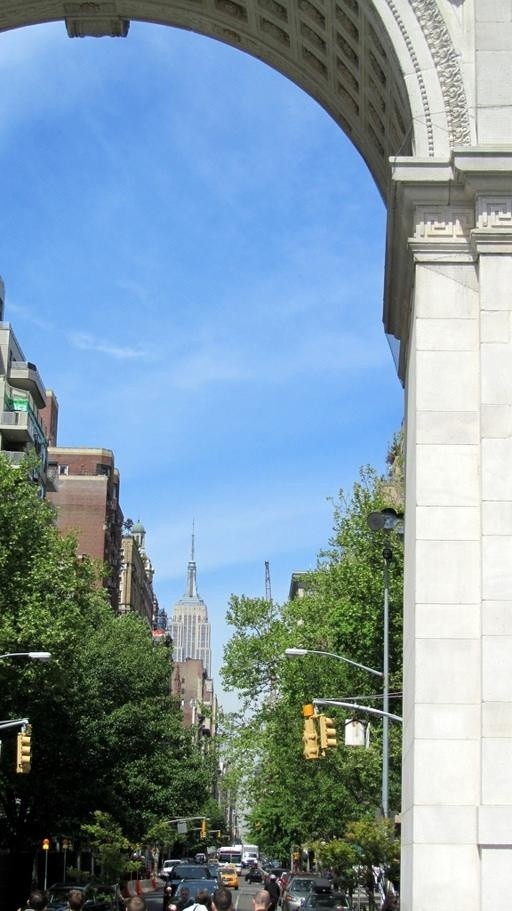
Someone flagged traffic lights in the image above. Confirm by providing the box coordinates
[41,837,53,853]
[16,733,33,776]
[318,715,338,751]
[202,831,206,839]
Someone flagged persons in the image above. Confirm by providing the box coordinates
[15,875,283,911]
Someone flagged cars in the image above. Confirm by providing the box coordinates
[40,877,125,911]
[166,877,221,911]
[276,871,400,910]
[160,853,290,891]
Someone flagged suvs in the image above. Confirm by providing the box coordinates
[163,863,223,907]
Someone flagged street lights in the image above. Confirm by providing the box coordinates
[283,508,405,820]
[1,649,53,667]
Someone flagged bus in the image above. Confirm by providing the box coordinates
[216,847,242,876]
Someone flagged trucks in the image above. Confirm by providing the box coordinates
[235,844,260,867]
